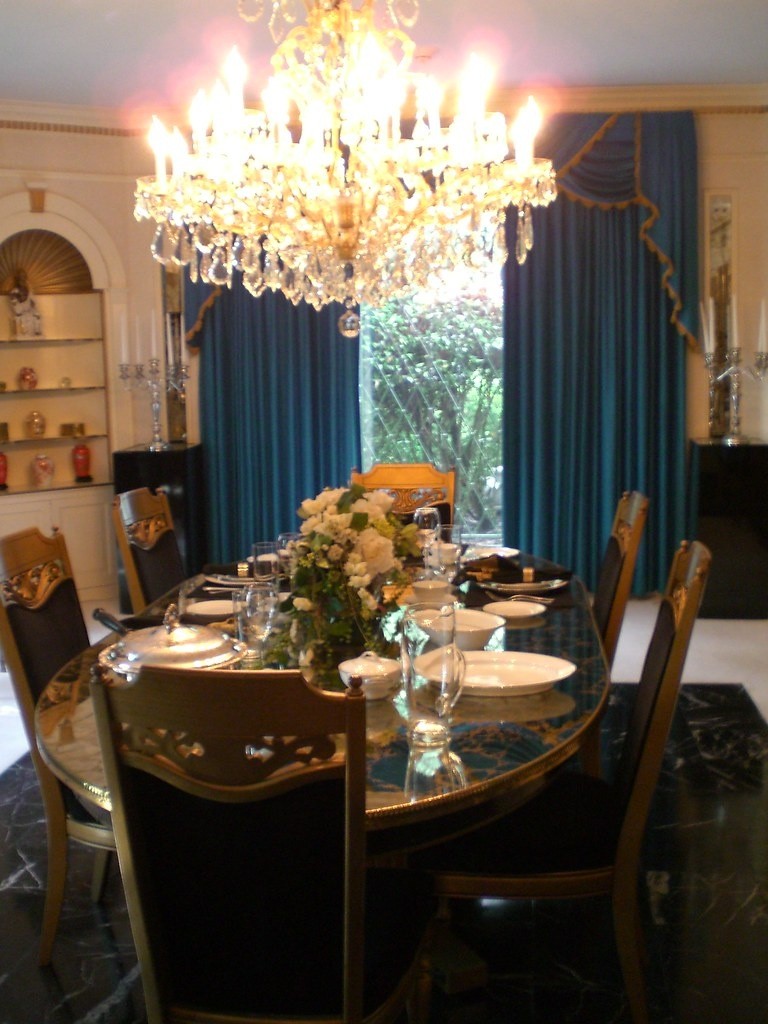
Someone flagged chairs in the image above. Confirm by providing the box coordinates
[87,663,436,1024]
[110,485,191,612]
[347,460,455,548]
[550,486,649,784]
[0,518,130,962]
[412,536,717,1024]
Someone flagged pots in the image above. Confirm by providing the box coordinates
[92,607,250,679]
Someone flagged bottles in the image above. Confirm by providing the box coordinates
[25,410,46,441]
[72,444,91,483]
[31,454,54,489]
[19,367,37,391]
[1,452,8,486]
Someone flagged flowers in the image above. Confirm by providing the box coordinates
[275,487,430,650]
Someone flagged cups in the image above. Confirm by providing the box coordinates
[438,524,462,583]
[230,590,264,670]
[398,601,457,748]
[277,533,302,569]
[253,542,281,619]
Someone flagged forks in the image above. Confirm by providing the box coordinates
[485,591,554,605]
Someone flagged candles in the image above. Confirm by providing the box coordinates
[167,310,173,366]
[179,314,187,366]
[757,295,768,355]
[150,308,158,361]
[708,298,715,354]
[137,315,142,364]
[701,301,709,355]
[119,311,128,365]
[725,294,739,352]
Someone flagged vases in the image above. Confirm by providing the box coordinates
[294,595,384,696]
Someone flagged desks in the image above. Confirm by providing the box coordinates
[36,540,609,1024]
[683,435,768,621]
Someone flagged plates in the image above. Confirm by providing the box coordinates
[412,650,578,698]
[185,599,248,623]
[483,601,547,619]
[476,580,568,595]
[471,546,521,559]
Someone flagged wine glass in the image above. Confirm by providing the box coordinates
[412,507,440,581]
[245,588,273,669]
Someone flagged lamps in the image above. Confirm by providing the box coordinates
[130,1,562,339]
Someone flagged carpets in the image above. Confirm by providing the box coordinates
[1,674,768,1024]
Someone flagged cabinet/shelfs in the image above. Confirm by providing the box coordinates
[113,442,206,613]
[0,291,121,640]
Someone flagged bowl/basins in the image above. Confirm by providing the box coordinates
[407,608,507,650]
[337,650,403,700]
[411,580,448,602]
